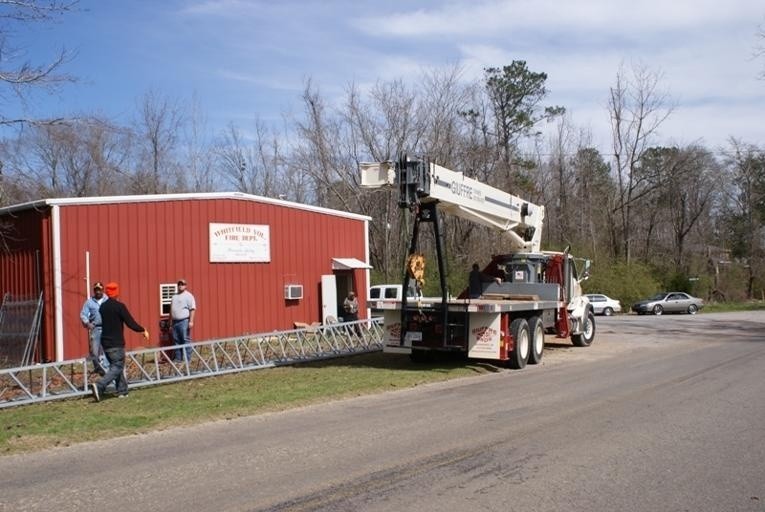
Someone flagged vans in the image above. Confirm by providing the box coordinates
[370,284,423,318]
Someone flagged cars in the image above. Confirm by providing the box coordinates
[584,294,622,316]
[632,292,705,315]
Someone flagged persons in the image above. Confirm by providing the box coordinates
[169,280,196,364]
[80,282,150,401]
[343,292,363,337]
[470,264,481,298]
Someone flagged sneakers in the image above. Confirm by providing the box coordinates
[118,394,128,398]
[91,383,99,401]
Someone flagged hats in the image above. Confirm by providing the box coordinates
[178,280,187,285]
[93,282,119,297]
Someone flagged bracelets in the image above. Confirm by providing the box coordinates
[169,325,172,327]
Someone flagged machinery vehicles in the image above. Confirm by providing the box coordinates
[357,151,596,369]
